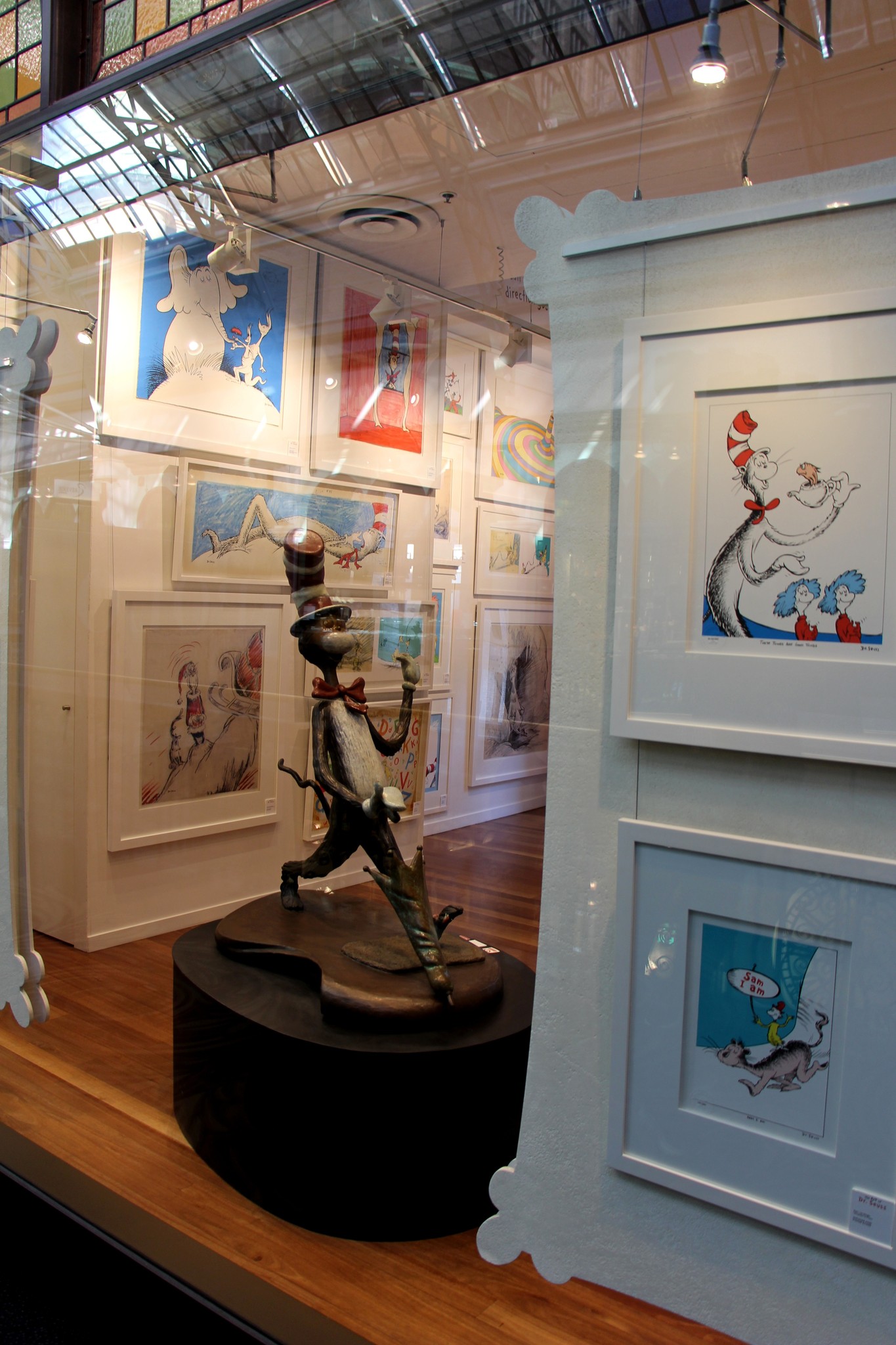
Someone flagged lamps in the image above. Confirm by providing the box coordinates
[498,321,533,368]
[207,214,246,275]
[0,293,98,345]
[368,271,403,327]
[689,0,786,186]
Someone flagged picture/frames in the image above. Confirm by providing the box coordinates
[96,199,312,469]
[420,433,471,565]
[603,817,896,1274]
[473,350,555,512]
[420,567,461,693]
[301,700,433,842]
[309,253,449,490]
[466,600,554,788]
[106,589,286,854]
[423,692,455,817]
[299,593,439,697]
[608,286,896,769]
[170,455,402,595]
[473,504,554,598]
